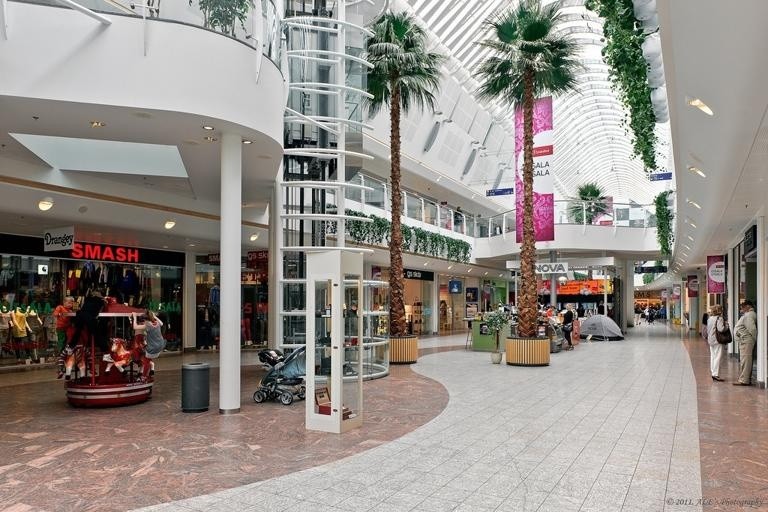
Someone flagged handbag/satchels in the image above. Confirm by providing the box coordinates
[564,323,573,332]
[716,328,733,345]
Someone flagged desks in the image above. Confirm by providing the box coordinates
[462,317,512,355]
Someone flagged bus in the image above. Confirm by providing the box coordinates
[207,283,305,341]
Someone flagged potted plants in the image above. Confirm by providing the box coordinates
[485,315,511,365]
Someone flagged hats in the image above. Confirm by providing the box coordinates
[739,298,754,306]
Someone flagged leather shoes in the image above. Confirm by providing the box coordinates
[732,380,751,385]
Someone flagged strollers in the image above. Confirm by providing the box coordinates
[252,345,306,405]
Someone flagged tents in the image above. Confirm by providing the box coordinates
[580,315,624,341]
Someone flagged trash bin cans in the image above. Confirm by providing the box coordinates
[181,362,210,413]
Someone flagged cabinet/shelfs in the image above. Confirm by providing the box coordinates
[301,251,364,434]
[539,316,563,353]
[412,303,425,336]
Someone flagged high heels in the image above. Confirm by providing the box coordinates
[711,375,726,382]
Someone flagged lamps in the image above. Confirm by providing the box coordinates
[161,216,176,232]
[39,197,55,211]
[249,231,261,243]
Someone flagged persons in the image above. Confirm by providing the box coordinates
[67,291,114,362]
[53,296,75,356]
[547,306,556,316]
[454,206,463,233]
[644,305,666,325]
[25,305,43,360]
[707,303,729,382]
[10,307,33,362]
[732,300,757,386]
[562,303,574,351]
[0,306,14,357]
[132,310,164,383]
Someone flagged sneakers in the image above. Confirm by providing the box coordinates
[64,345,73,357]
[102,353,115,363]
[566,346,574,350]
[57,372,64,379]
